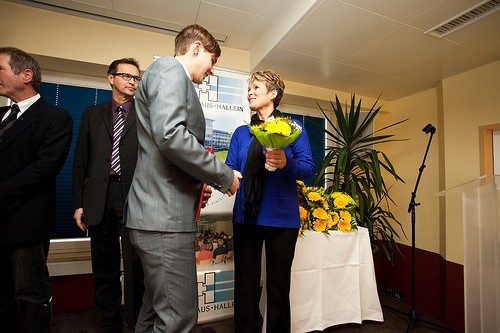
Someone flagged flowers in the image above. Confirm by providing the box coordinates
[295,180,358,236]
[243,115,302,172]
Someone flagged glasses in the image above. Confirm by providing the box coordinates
[113,72,141,83]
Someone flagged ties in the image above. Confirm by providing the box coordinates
[110,105,125,177]
[1,102,18,135]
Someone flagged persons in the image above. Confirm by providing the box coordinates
[72,58,140,333]
[0,47,72,333]
[226,70,316,333]
[124,25,240,333]
[195,228,233,264]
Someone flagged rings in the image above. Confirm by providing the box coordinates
[279,160,281,163]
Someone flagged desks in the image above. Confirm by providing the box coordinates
[259,225,384,333]
